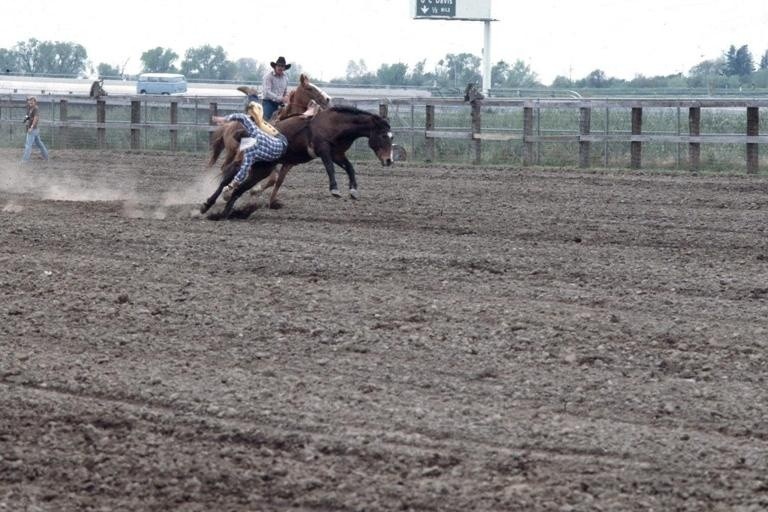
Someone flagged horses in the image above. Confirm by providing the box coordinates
[199,73,394,222]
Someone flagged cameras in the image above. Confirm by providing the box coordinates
[21,115,29,123]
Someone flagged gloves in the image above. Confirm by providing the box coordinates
[222,179,239,201]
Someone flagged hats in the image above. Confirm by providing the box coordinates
[271,57,291,70]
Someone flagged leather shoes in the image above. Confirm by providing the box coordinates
[238,86,256,94]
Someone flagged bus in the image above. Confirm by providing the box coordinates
[137,73,188,96]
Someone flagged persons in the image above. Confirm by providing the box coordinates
[261,57,291,120]
[212,86,290,202]
[21,97,49,164]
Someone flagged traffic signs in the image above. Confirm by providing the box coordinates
[416,0,457,17]
